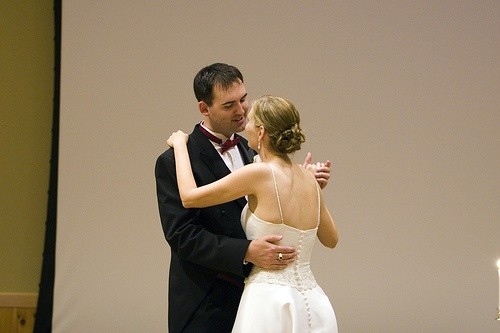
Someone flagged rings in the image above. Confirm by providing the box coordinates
[278,252,283,261]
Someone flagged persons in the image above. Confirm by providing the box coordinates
[154,61,332,332]
[165,94,338,332]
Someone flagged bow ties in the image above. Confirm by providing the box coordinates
[199,121,241,154]
